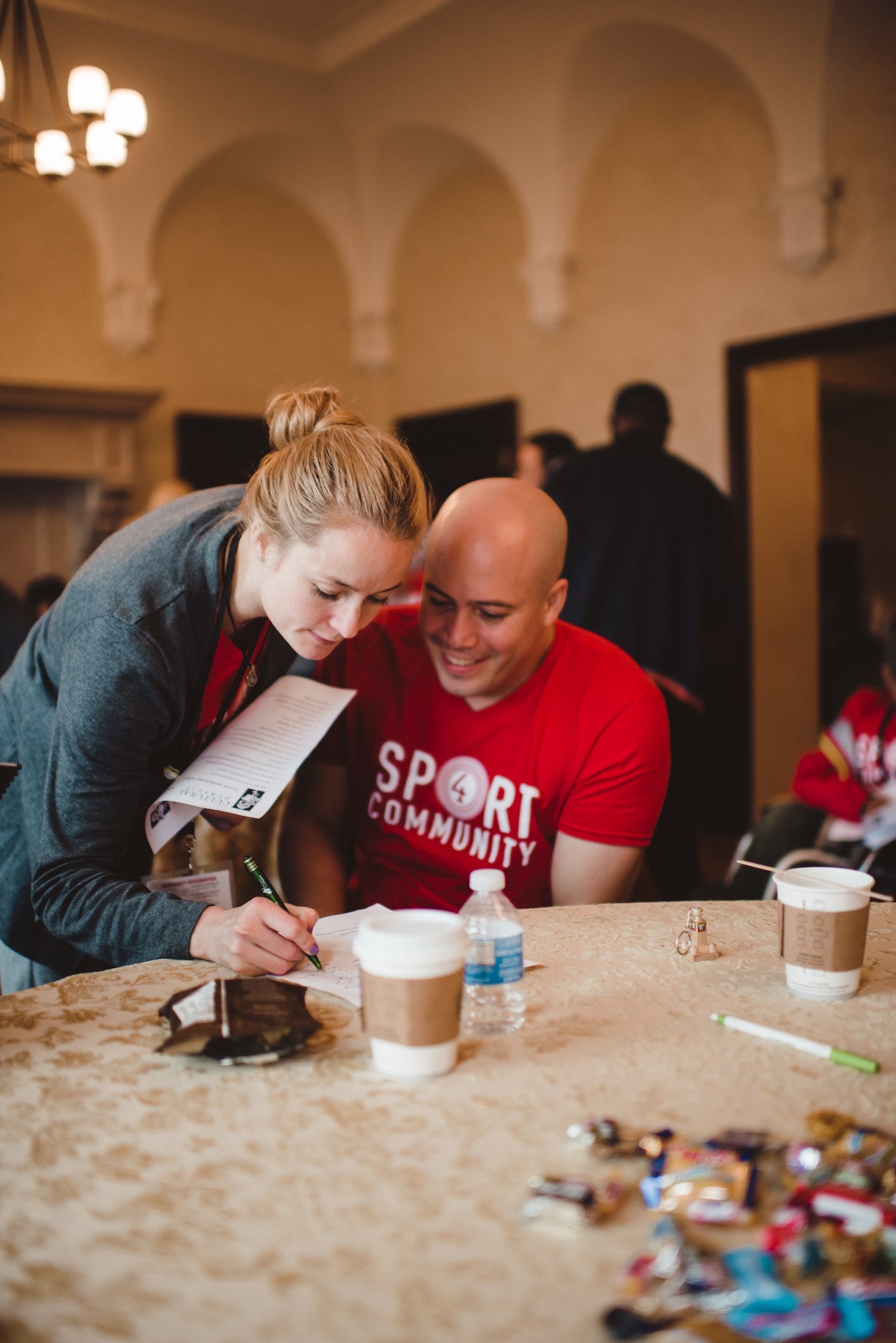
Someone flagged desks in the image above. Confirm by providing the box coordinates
[0,889,896,1343]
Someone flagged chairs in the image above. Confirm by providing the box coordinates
[721,799,881,906]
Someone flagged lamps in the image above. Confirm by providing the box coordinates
[0,0,152,199]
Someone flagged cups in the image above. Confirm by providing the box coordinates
[772,867,875,1000]
[353,909,471,1079]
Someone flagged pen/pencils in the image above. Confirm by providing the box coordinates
[707,1007,882,1078]
[243,853,322,976]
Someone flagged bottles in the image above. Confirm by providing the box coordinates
[458,869,528,1037]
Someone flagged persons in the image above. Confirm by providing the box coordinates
[276,475,672,926]
[0,382,432,993]
[0,381,895,1011]
